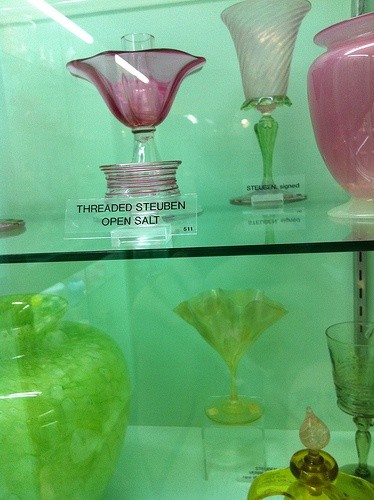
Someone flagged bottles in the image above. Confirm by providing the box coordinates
[0,293,132,500]
[246,406,374,500]
[305,10,374,219]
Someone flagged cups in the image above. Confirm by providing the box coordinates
[121,32,155,52]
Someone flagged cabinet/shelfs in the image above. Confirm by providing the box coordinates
[0,0,374,500]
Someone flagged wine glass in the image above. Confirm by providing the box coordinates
[219,0,312,206]
[323,320,374,486]
[64,49,206,163]
[172,288,288,425]
